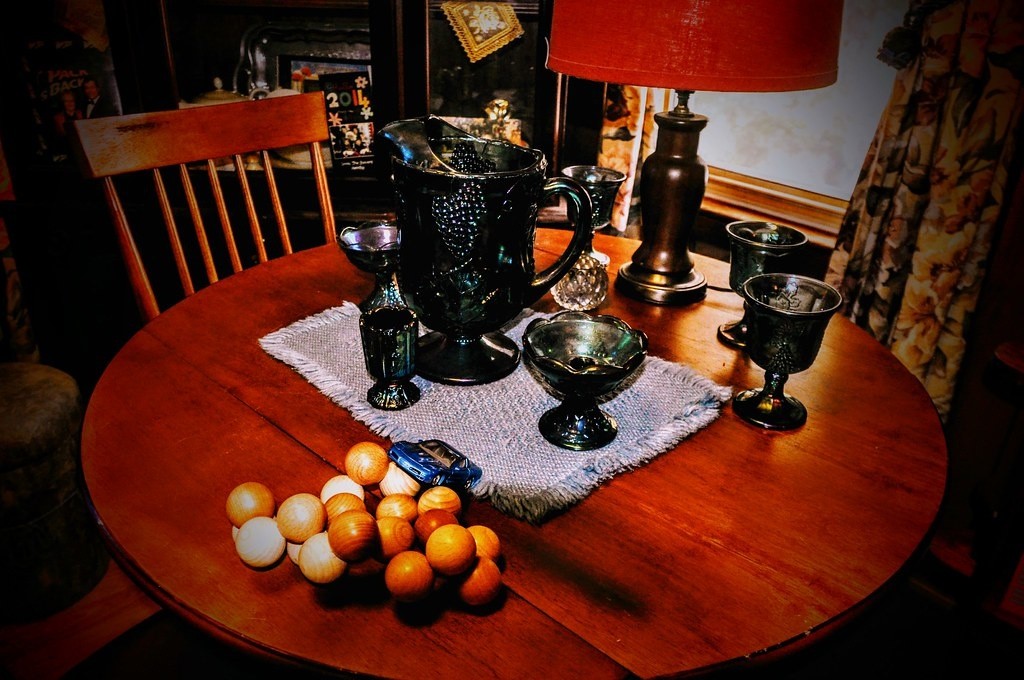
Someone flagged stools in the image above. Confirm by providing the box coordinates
[0,362,105,624]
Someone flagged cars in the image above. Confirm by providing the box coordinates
[387,438,483,492]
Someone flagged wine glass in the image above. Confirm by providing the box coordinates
[522,312,648,452]
[717,220,809,348]
[359,305,421,410]
[732,273,842,430]
[337,221,405,311]
[561,165,627,265]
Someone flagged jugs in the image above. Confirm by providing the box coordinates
[369,117,592,386]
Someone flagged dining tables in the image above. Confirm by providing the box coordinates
[82,232,951,680]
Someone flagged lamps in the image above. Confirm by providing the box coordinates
[547,0,844,305]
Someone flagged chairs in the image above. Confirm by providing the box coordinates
[69,90,337,323]
